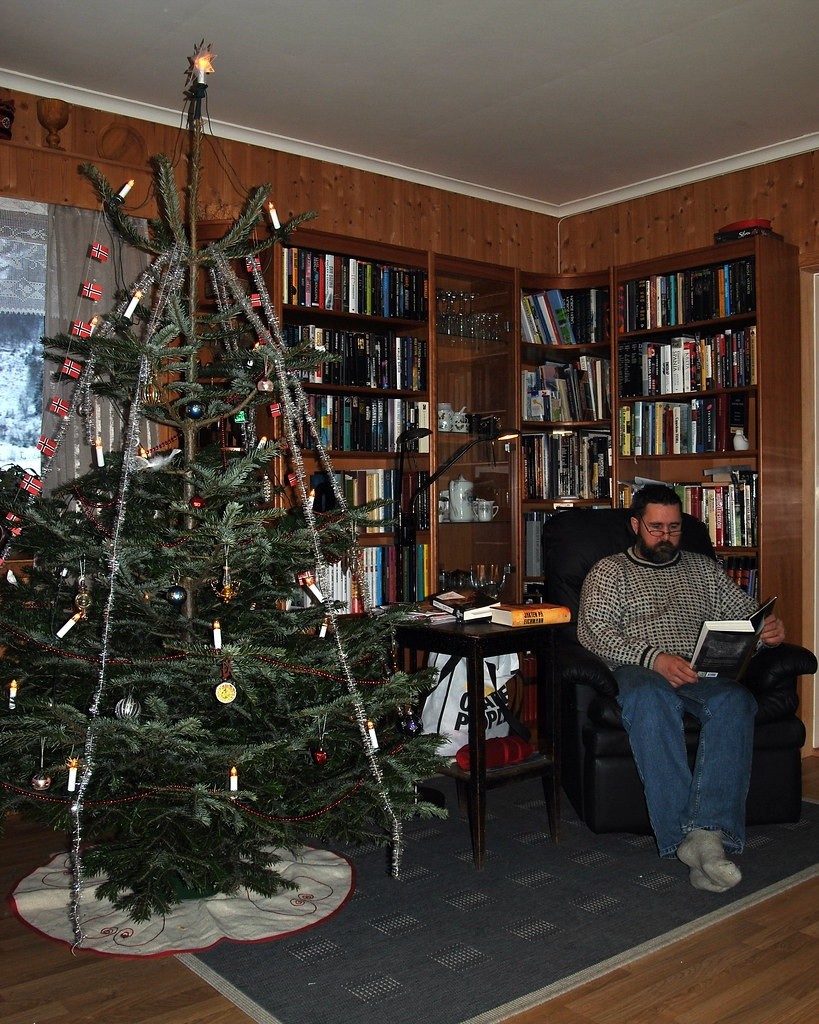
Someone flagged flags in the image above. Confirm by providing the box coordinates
[6,242,109,535]
[246,258,296,486]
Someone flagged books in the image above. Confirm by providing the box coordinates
[428,585,501,621]
[617,259,756,455]
[281,247,429,454]
[490,603,571,628]
[690,596,777,680]
[617,474,759,600]
[521,289,613,501]
[288,469,430,615]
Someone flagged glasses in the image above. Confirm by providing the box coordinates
[641,516,682,537]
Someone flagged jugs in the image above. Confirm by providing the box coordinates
[450,476,474,522]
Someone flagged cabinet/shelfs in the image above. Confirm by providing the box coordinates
[195,219,799,756]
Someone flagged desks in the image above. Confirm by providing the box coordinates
[380,618,571,861]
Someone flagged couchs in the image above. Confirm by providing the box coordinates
[538,509,818,833]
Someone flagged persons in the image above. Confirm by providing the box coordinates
[577,486,786,894]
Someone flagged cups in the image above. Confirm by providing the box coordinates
[438,410,453,432]
[438,501,444,523]
[466,414,481,433]
[472,501,499,521]
[452,412,469,433]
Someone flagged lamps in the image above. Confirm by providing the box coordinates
[409,427,522,675]
[396,424,432,672]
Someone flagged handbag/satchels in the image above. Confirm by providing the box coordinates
[414,651,520,756]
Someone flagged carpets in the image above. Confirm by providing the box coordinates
[167,761,814,1024]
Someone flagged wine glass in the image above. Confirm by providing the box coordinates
[436,289,500,340]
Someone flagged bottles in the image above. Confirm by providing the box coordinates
[440,563,499,594]
